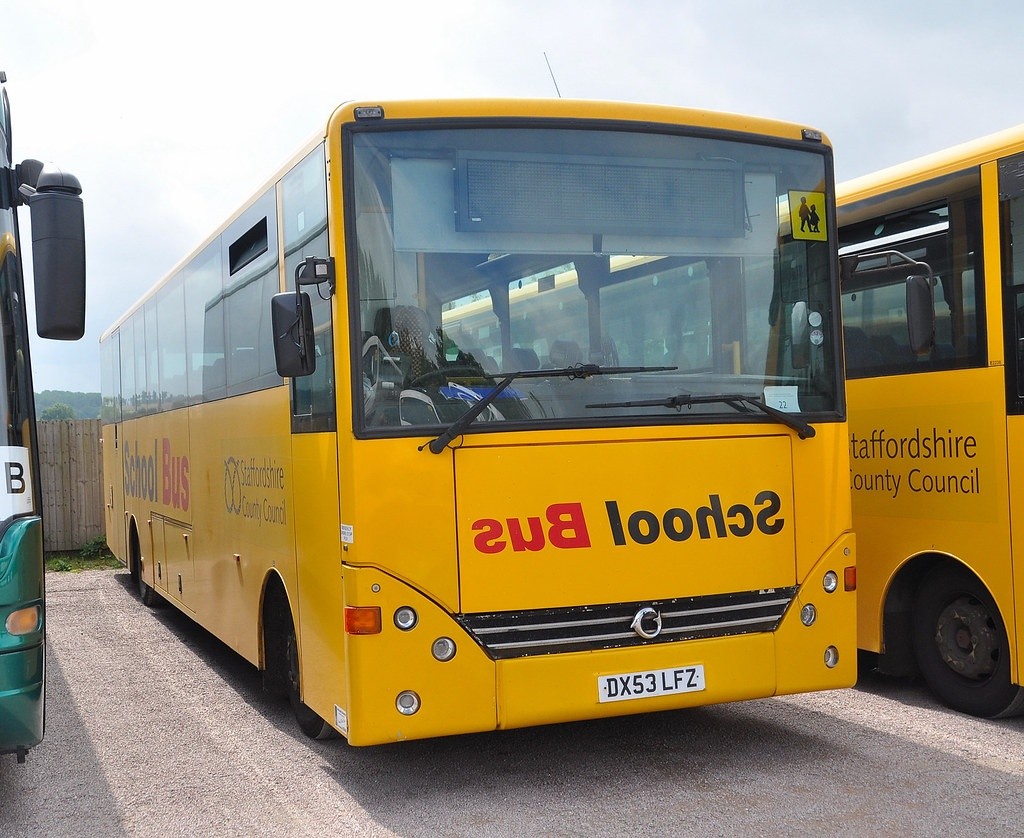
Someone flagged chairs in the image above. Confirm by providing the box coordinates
[454,341,584,374]
[368,303,441,388]
[842,324,903,368]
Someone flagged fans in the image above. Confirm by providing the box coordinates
[588,334,619,368]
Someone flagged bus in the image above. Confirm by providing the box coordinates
[95,99,1024,745]
[0,74,88,764]
